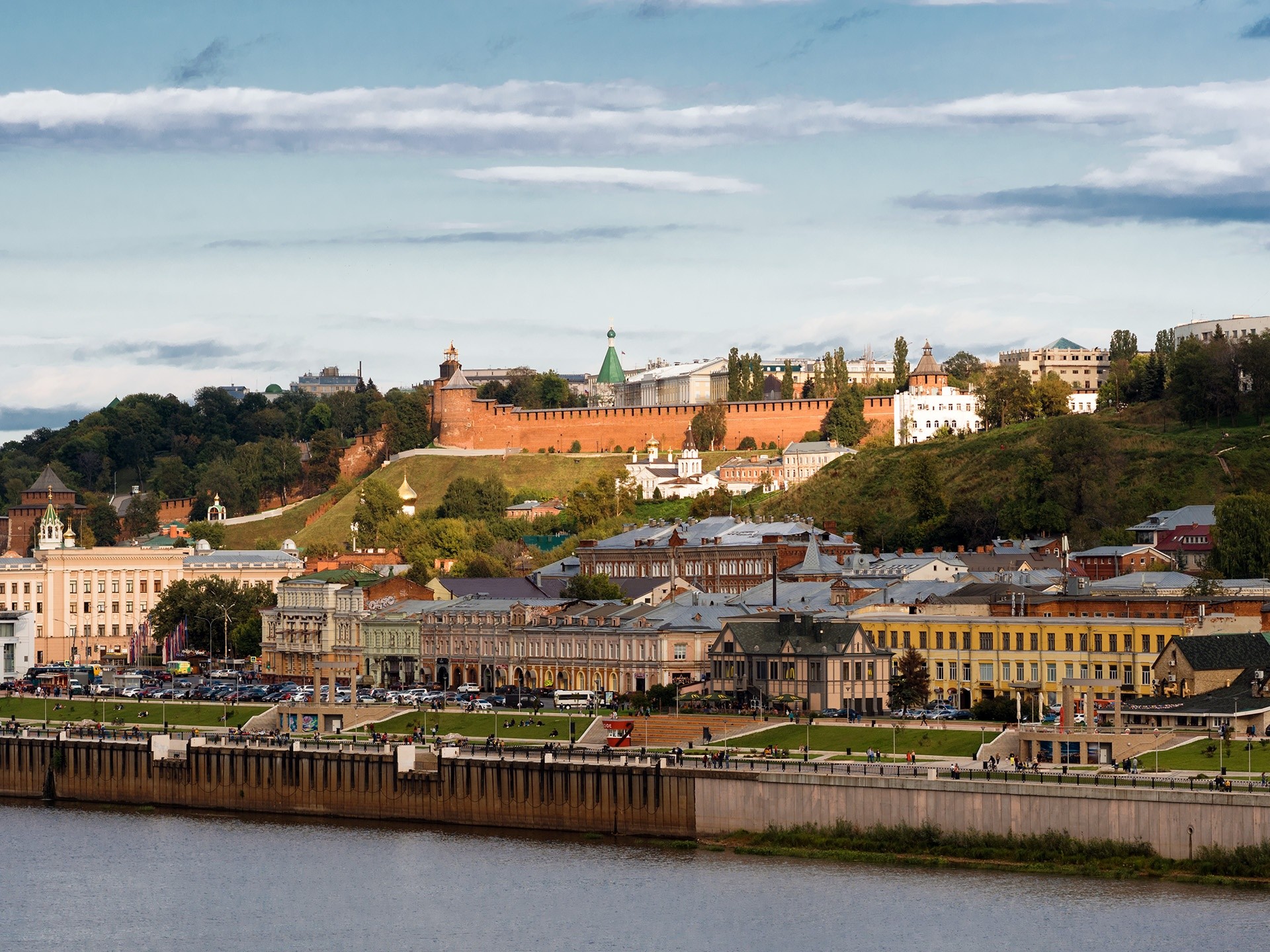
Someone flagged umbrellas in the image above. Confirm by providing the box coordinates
[1017,692,1044,728]
[1084,693,1087,726]
[672,692,809,709]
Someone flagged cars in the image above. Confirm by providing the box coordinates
[815,708,839,717]
[831,708,860,718]
[890,702,1089,729]
[0,660,605,709]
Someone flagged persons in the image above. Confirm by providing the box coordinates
[190,662,314,685]
[363,694,651,759]
[0,659,164,736]
[191,728,322,748]
[1122,757,1138,774]
[680,701,861,724]
[1151,717,1156,727]
[667,743,809,769]
[1214,776,1233,792]
[920,713,928,726]
[950,750,1081,780]
[866,747,916,765]
[285,692,312,707]
[221,714,225,722]
[232,695,235,705]
[1221,723,1259,740]
[1261,772,1266,786]
[358,696,361,706]
[327,681,329,685]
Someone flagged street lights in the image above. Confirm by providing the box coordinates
[44,696,47,729]
[288,704,291,740]
[723,719,727,755]
[1218,732,1223,775]
[354,706,359,741]
[1247,738,1252,780]
[981,726,986,770]
[1066,730,1071,774]
[806,721,810,761]
[568,712,573,745]
[102,697,105,724]
[223,701,227,732]
[1154,733,1161,777]
[645,715,649,752]
[494,710,498,743]
[1233,697,1239,739]
[162,699,166,728]
[54,603,236,665]
[66,666,1035,735]
[423,709,428,745]
[891,723,897,766]
[1097,729,1102,769]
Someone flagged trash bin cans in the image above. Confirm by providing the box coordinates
[810,715,813,724]
[983,761,988,769]
[571,736,575,743]
[1003,724,1007,731]
[164,728,167,734]
[569,744,573,751]
[804,754,807,760]
[165,721,167,727]
[972,753,977,760]
[42,723,45,729]
[1062,765,1068,774]
[703,726,709,738]
[955,772,960,780]
[847,748,851,755]
[589,710,593,716]
[1222,767,1226,775]
[641,747,645,754]
[571,722,575,733]
[764,716,768,721]
[491,734,494,738]
[688,741,693,748]
[238,724,241,729]
[1112,759,1116,765]
[11,715,15,720]
[353,736,356,741]
[871,720,876,727]
[422,738,425,744]
[336,728,340,735]
[720,750,725,758]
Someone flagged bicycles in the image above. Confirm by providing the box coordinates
[111,718,125,726]
